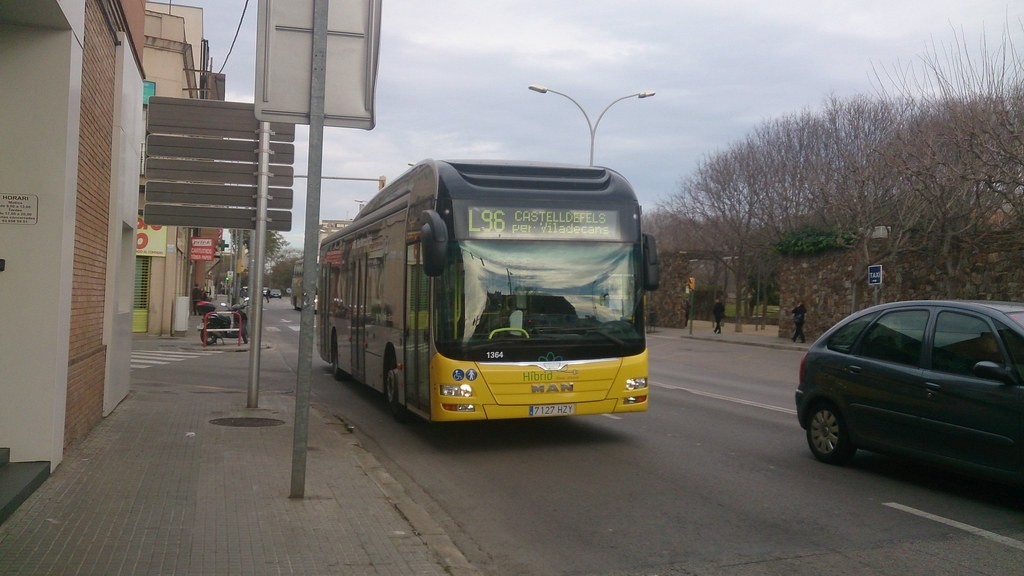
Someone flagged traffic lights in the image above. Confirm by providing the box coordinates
[225,277,230,282]
[689,277,695,290]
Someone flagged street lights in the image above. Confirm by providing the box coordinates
[526,83,656,166]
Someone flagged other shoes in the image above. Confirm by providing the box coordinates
[801,340,806,343]
[792,338,796,342]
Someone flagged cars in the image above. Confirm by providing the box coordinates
[794,300,1024,495]
[263,287,269,296]
[269,288,282,299]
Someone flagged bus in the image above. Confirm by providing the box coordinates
[290,259,320,311]
[322,158,661,423]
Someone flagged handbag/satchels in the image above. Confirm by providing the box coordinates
[795,314,802,324]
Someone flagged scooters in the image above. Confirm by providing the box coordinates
[195,296,252,345]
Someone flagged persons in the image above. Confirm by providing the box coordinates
[970,330,1002,363]
[713,298,724,334]
[791,301,806,343]
[266,289,270,303]
[192,284,201,316]
